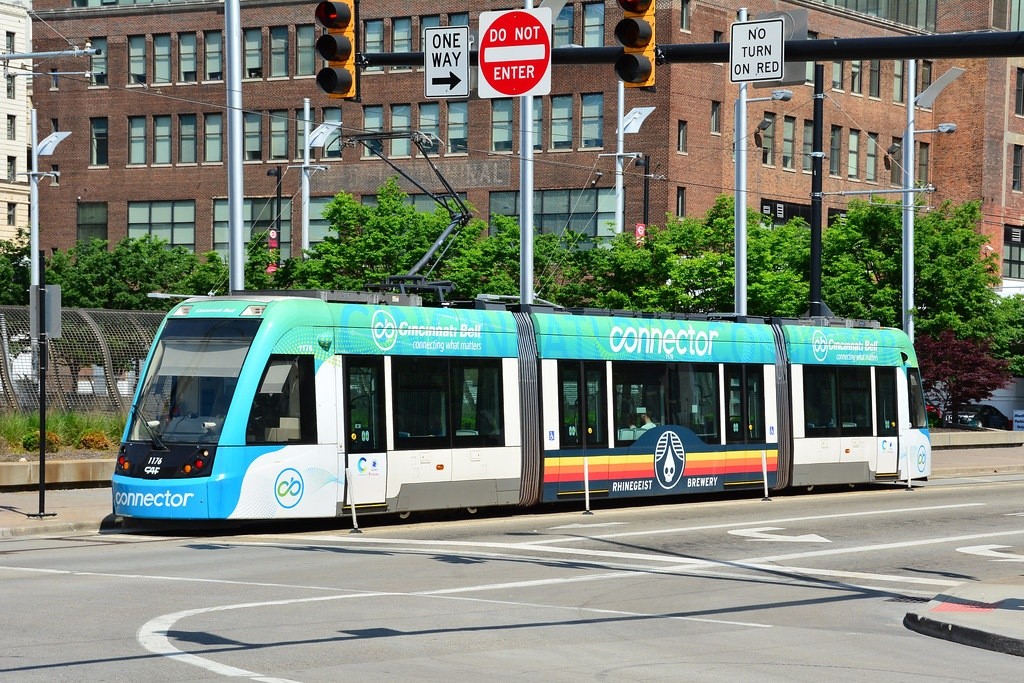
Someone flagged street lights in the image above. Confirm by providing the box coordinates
[266,164,282,270]
[636,155,650,245]
[903,60,956,345]
[730,6,793,316]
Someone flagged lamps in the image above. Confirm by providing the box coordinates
[888,143,901,154]
[754,118,772,134]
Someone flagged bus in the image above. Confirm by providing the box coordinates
[111,127,936,521]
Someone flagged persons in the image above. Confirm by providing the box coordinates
[626,407,656,430]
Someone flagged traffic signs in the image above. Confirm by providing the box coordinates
[423,26,472,100]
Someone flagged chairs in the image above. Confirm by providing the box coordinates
[731,416,743,440]
[564,422,576,445]
[456,430,479,436]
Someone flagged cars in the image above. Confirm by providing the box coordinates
[943,404,1009,430]
[925,398,942,420]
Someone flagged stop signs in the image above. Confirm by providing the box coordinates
[476,6,553,97]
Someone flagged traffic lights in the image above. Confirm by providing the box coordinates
[614,0,656,88]
[313,0,359,98]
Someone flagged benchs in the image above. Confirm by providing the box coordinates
[618,428,647,441]
